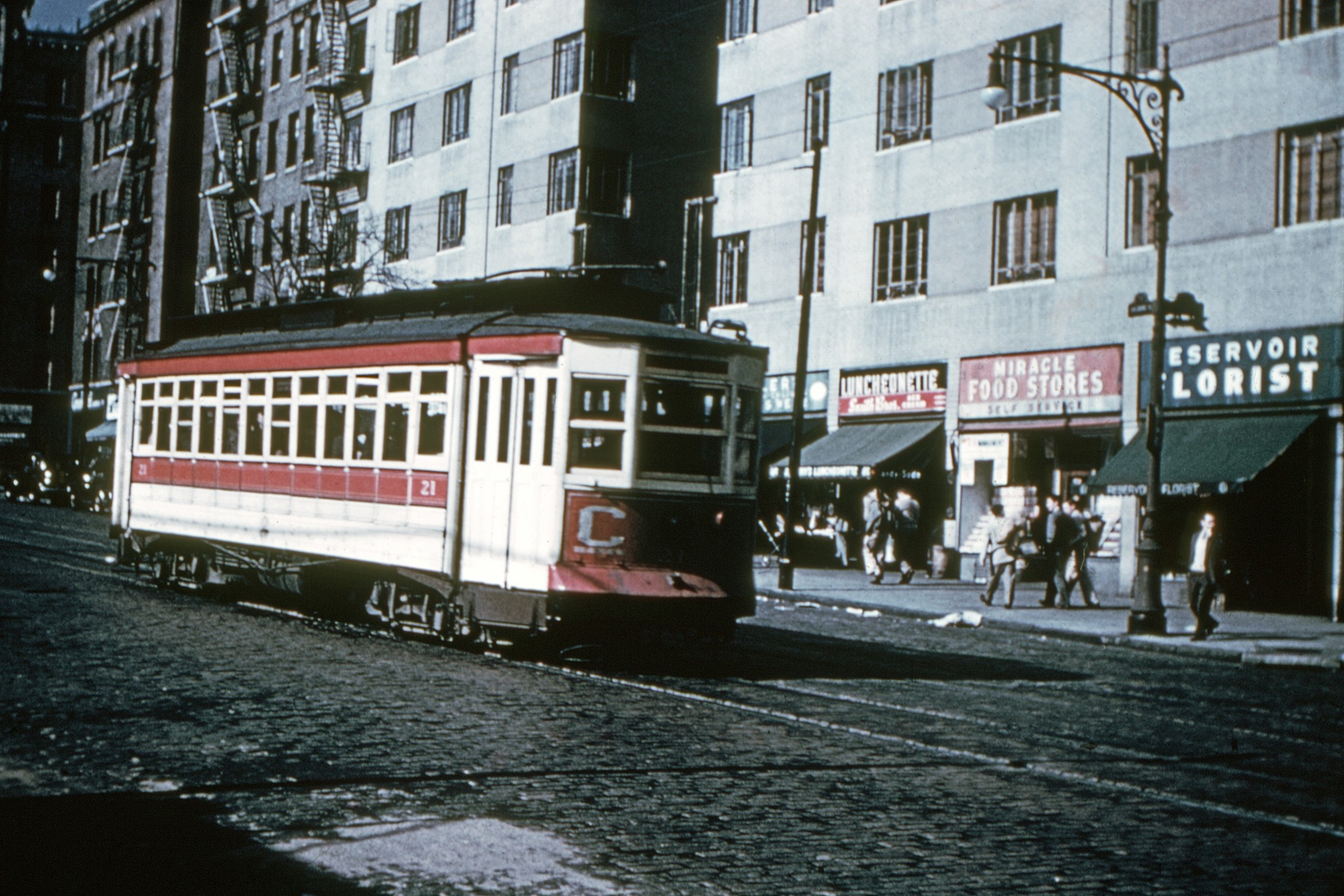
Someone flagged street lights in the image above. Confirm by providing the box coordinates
[980,41,1183,637]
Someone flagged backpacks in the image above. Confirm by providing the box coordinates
[1076,510,1103,550]
[1049,513,1078,550]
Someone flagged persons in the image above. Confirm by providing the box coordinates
[830,506,852,567]
[1055,500,1100,607]
[1032,494,1079,608]
[887,488,920,584]
[978,503,1020,608]
[861,484,889,585]
[1184,511,1229,641]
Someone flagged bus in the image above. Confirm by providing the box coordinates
[105,279,770,661]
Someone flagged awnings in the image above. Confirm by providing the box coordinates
[769,420,946,480]
[760,419,824,459]
[1077,411,1322,495]
[85,420,117,443]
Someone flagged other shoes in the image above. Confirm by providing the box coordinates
[868,568,1103,609]
[1208,620,1220,635]
[1193,630,1209,642]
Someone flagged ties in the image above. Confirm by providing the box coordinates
[1201,531,1207,541]
